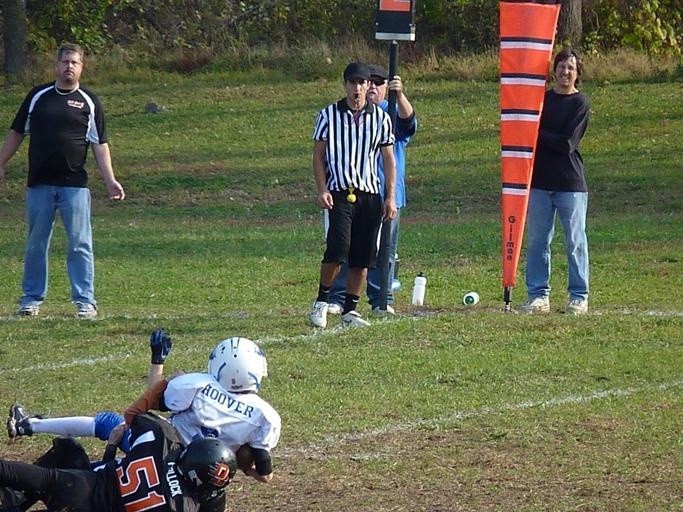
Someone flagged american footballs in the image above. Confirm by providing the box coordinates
[236,445,253,471]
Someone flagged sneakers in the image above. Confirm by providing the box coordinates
[19,305,40,316]
[75,303,98,321]
[564,298,589,315]
[372,305,397,317]
[340,309,371,329]
[7,403,43,438]
[518,296,551,315]
[309,299,341,329]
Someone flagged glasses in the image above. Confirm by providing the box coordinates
[369,77,387,86]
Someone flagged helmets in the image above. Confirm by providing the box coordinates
[207,336,269,394]
[177,437,238,494]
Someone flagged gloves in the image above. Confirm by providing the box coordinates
[150,328,172,365]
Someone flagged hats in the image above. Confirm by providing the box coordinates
[368,64,389,80]
[344,63,371,81]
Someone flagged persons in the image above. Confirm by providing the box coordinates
[525,48,590,315]
[311,63,398,330]
[0,45,125,320]
[327,65,417,316]
[0,380,238,512]
[7,330,281,484]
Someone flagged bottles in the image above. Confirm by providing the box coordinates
[410,270,428,306]
[462,291,479,306]
